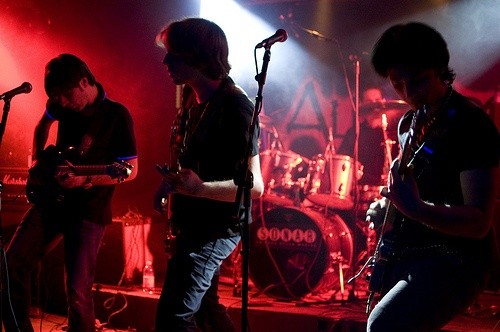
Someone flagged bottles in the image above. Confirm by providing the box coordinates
[142,261,155,295]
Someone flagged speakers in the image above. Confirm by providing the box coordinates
[92,216,154,287]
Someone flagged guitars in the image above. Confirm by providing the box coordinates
[366,103,431,294]
[154,161,181,255]
[24,145,132,206]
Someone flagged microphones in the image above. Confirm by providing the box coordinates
[0,82,32,100]
[256,28,288,48]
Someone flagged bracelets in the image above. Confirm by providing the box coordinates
[83,176,92,189]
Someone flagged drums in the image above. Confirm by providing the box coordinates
[257,150,312,208]
[248,205,354,301]
[354,184,387,224]
[304,153,364,209]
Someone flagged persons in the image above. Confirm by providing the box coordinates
[0,53,138,332]
[339,88,385,185]
[153,17,265,332]
[367,22,500,332]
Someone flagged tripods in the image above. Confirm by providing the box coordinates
[294,50,379,312]
